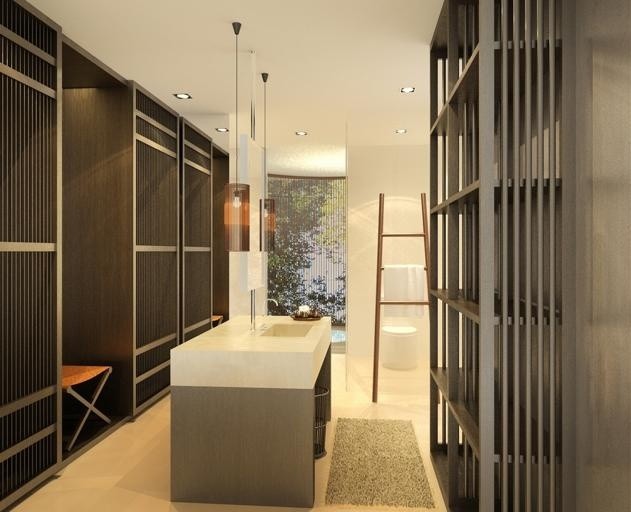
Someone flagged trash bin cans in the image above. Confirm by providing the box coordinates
[313,386,329,459]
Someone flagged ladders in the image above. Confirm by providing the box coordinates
[373,193,441,404]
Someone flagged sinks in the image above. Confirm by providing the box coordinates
[259,322,314,337]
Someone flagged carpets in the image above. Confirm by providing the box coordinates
[325,417,434,509]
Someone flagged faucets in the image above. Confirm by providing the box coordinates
[263,298,278,328]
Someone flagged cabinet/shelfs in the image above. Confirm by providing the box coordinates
[426,0,593,512]
[0,0,230,512]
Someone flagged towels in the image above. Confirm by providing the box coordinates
[383,263,424,318]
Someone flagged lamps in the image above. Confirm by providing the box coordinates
[262,73,268,218]
[232,22,241,210]
